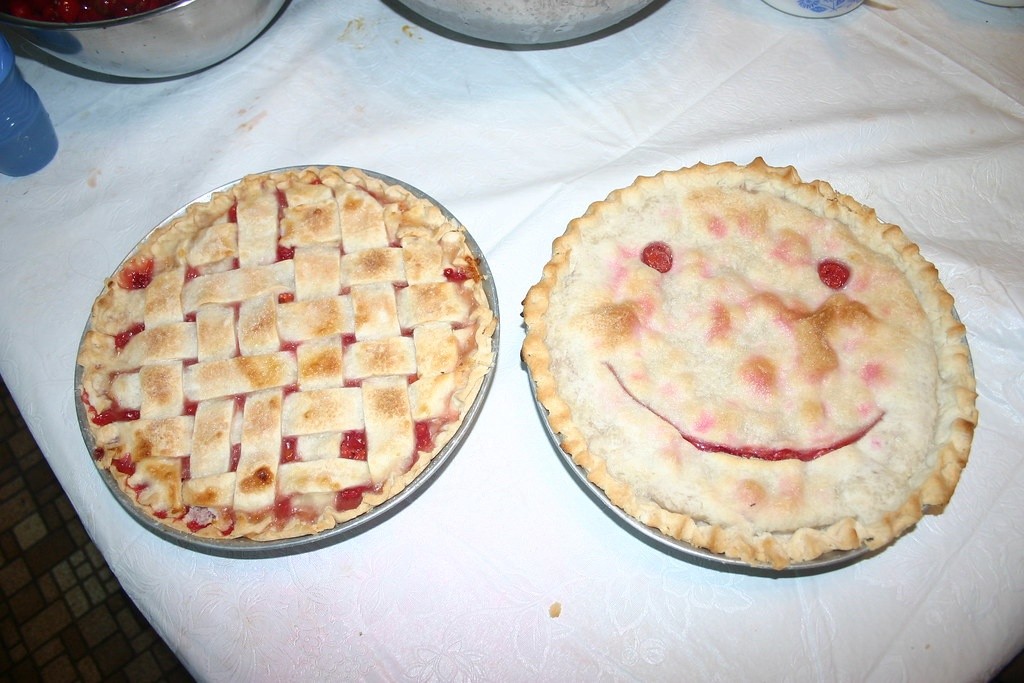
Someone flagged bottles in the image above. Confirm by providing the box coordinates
[0,35,59,178]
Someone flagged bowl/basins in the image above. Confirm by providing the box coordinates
[0,0,288,80]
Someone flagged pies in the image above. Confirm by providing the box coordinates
[76,165,498,544]
[519,157,979,568]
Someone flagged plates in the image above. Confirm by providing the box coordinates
[73,163,501,559]
[527,306,977,571]
[399,0,655,46]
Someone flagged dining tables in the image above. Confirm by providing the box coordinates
[2,0,1024,683]
